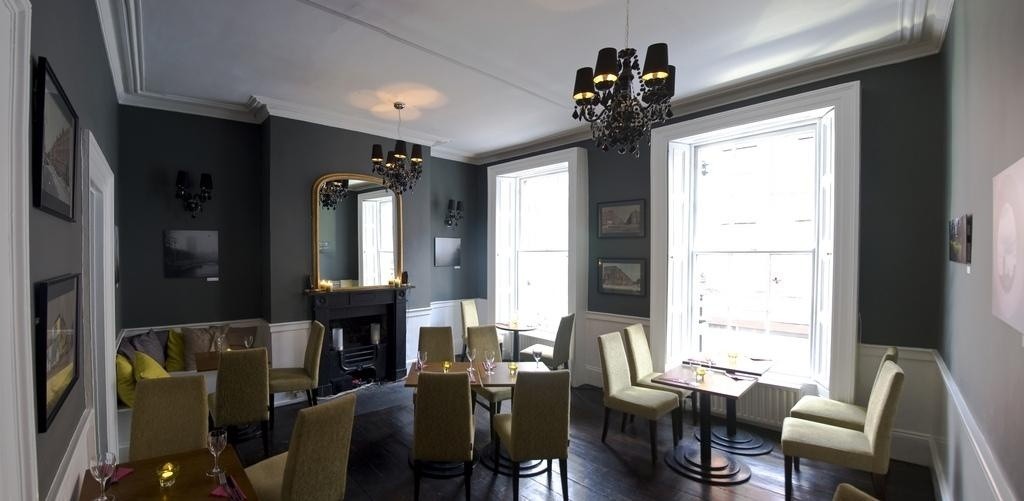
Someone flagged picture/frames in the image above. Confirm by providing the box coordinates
[32,273,82,435]
[596,256,648,298]
[29,54,81,227]
[595,198,646,240]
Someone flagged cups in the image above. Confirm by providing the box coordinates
[482,361,496,376]
[156,462,177,489]
[441,361,451,370]
[325,284,333,293]
[388,281,393,287]
[508,366,516,377]
[394,280,400,289]
[242,336,253,349]
[320,283,327,291]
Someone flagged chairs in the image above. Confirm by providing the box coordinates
[466,325,499,363]
[125,375,209,464]
[518,314,578,371]
[406,367,480,500]
[456,299,503,362]
[830,482,878,501]
[242,390,358,500]
[205,347,271,461]
[779,361,904,501]
[790,345,899,490]
[415,326,456,364]
[598,331,678,468]
[485,367,575,501]
[626,321,699,445]
[264,318,324,432]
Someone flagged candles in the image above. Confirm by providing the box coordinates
[393,278,401,289]
[387,278,394,286]
[319,279,326,291]
[325,281,333,293]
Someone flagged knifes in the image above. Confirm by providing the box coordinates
[226,473,248,501]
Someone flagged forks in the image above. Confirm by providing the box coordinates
[216,470,235,500]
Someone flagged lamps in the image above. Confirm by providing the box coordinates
[318,180,350,212]
[369,100,423,195]
[172,169,216,219]
[570,0,678,160]
[444,199,463,229]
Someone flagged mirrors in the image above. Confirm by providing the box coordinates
[310,174,403,290]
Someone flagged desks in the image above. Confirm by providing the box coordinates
[401,359,554,479]
[500,321,538,361]
[71,437,259,500]
[646,364,757,490]
[689,351,784,456]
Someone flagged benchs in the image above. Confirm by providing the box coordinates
[111,318,275,452]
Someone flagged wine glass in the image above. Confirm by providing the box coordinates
[204,430,228,478]
[532,350,542,372]
[484,350,495,379]
[686,359,695,377]
[417,350,427,376]
[465,346,476,372]
[88,452,116,501]
[216,336,224,352]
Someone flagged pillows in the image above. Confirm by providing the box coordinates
[112,322,256,409]
